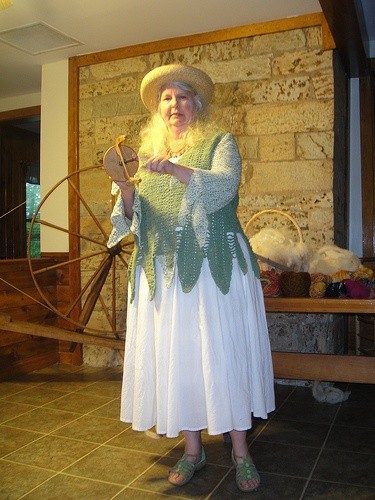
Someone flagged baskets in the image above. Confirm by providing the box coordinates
[244,209,306,274]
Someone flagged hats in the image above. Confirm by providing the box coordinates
[139,63,215,113]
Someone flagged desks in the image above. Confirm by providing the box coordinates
[262,297,375,385]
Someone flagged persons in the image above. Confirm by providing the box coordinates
[105,63,277,492]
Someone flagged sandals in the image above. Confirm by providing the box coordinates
[168,445,207,485]
[231,447,261,490]
